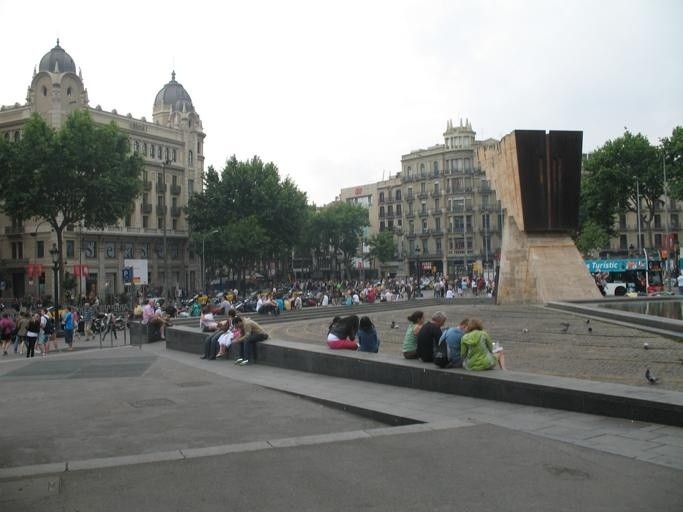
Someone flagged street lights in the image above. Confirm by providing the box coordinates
[50,243,65,337]
[415,244,423,297]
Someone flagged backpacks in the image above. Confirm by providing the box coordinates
[433,330,452,365]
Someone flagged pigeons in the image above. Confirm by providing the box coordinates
[586,320,590,325]
[643,342,649,350]
[588,327,592,333]
[523,328,529,334]
[560,323,570,334]
[391,320,399,329]
[645,369,662,385]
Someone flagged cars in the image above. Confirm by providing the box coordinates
[42,306,96,337]
[235,296,257,312]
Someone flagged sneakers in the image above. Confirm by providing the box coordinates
[235,358,248,365]
[215,352,223,358]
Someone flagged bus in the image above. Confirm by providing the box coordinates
[585,257,664,296]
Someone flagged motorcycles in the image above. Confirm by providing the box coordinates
[100,313,125,331]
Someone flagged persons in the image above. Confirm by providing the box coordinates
[255,277,421,318]
[676,273,683,294]
[197,306,270,364]
[594,271,606,297]
[132,288,238,339]
[417,270,496,300]
[1,292,119,358]
[403,310,506,371]
[326,313,380,354]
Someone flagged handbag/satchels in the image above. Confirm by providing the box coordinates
[41,315,52,334]
